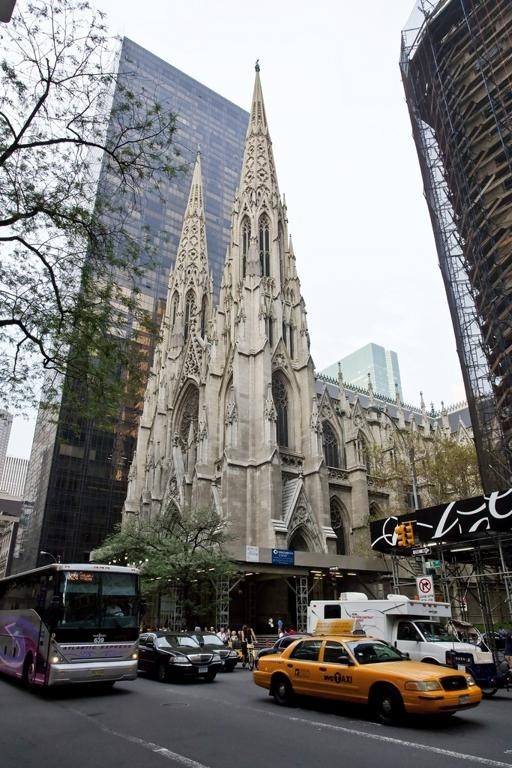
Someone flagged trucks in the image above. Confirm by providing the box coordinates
[306,592,482,665]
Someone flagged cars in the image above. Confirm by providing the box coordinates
[253,619,483,726]
[478,631,506,651]
[138,631,238,683]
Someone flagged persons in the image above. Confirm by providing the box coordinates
[140,624,258,667]
[500,626,512,669]
[268,616,304,639]
[107,600,127,617]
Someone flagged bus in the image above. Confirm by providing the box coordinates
[0,563,141,694]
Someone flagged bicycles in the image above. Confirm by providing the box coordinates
[243,641,257,671]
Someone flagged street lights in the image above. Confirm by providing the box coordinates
[366,403,435,637]
[40,551,60,563]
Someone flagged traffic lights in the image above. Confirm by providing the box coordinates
[395,525,415,547]
